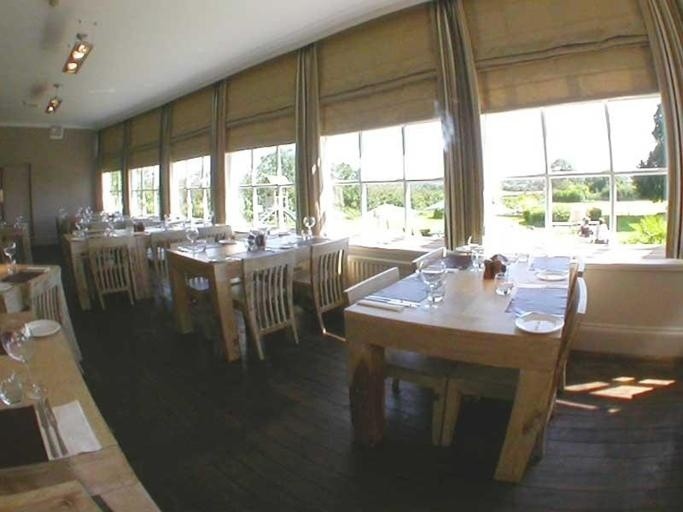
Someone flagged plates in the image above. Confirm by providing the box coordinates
[515,312,565,334]
[280,243,294,248]
[536,270,569,281]
[217,239,237,245]
[22,267,50,273]
[19,319,60,337]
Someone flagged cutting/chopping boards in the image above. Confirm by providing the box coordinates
[1,480,104,512]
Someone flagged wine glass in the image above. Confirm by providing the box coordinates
[421,260,447,311]
[2,242,17,274]
[185,227,200,256]
[467,234,488,274]
[498,251,518,288]
[0,369,26,407]
[0,319,49,400]
[303,216,316,241]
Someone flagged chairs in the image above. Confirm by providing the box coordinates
[341,266,441,447]
[28,267,65,328]
[558,251,586,395]
[293,235,351,335]
[232,248,305,362]
[411,246,447,272]
[15,231,41,265]
[150,230,194,301]
[87,237,137,311]
[440,275,596,460]
[197,224,233,241]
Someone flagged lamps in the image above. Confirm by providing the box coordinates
[44,83,63,116]
[61,33,95,76]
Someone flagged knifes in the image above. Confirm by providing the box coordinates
[366,295,420,309]
[65,459,114,512]
[43,398,69,456]
[37,404,60,458]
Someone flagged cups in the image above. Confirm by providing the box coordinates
[6,259,17,275]
[495,273,513,296]
[301,230,311,242]
[517,253,532,264]
[205,243,225,260]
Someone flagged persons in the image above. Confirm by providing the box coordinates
[578,217,609,244]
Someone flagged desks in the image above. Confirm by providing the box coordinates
[0,221,31,264]
[62,227,226,312]
[343,251,572,476]
[0,263,83,365]
[0,311,162,512]
[166,232,335,364]
